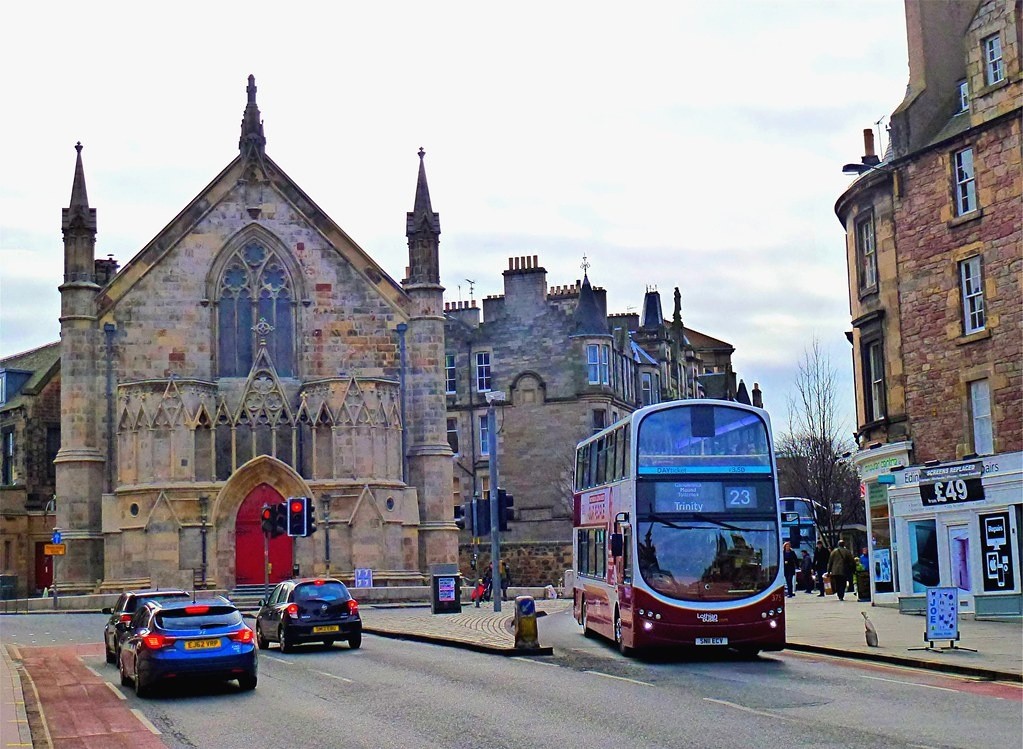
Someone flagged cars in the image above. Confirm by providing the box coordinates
[256,578,363,653]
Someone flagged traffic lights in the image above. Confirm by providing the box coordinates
[497,487,514,531]
[287,497,306,536]
[277,501,287,530]
[262,505,272,532]
[455,503,472,530]
[307,498,316,537]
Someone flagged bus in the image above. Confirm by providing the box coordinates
[779,496,830,566]
[573,393,800,656]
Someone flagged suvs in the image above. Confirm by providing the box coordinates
[115,596,260,697]
[101,588,193,668]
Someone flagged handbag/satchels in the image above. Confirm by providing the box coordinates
[847,566,853,578]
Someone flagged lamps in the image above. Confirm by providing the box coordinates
[890,465,906,471]
[869,443,883,449]
[925,459,940,467]
[843,452,852,458]
[962,452,978,460]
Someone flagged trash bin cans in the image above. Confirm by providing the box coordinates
[431,573,462,613]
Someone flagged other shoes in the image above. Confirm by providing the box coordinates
[818,594,824,596]
[805,591,811,593]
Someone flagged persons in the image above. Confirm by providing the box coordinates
[846,560,856,592]
[500,563,513,601]
[731,442,764,466]
[783,542,800,598]
[827,540,852,601]
[852,557,865,596]
[811,541,830,597]
[860,547,869,570]
[800,550,812,590]
[483,561,492,602]
